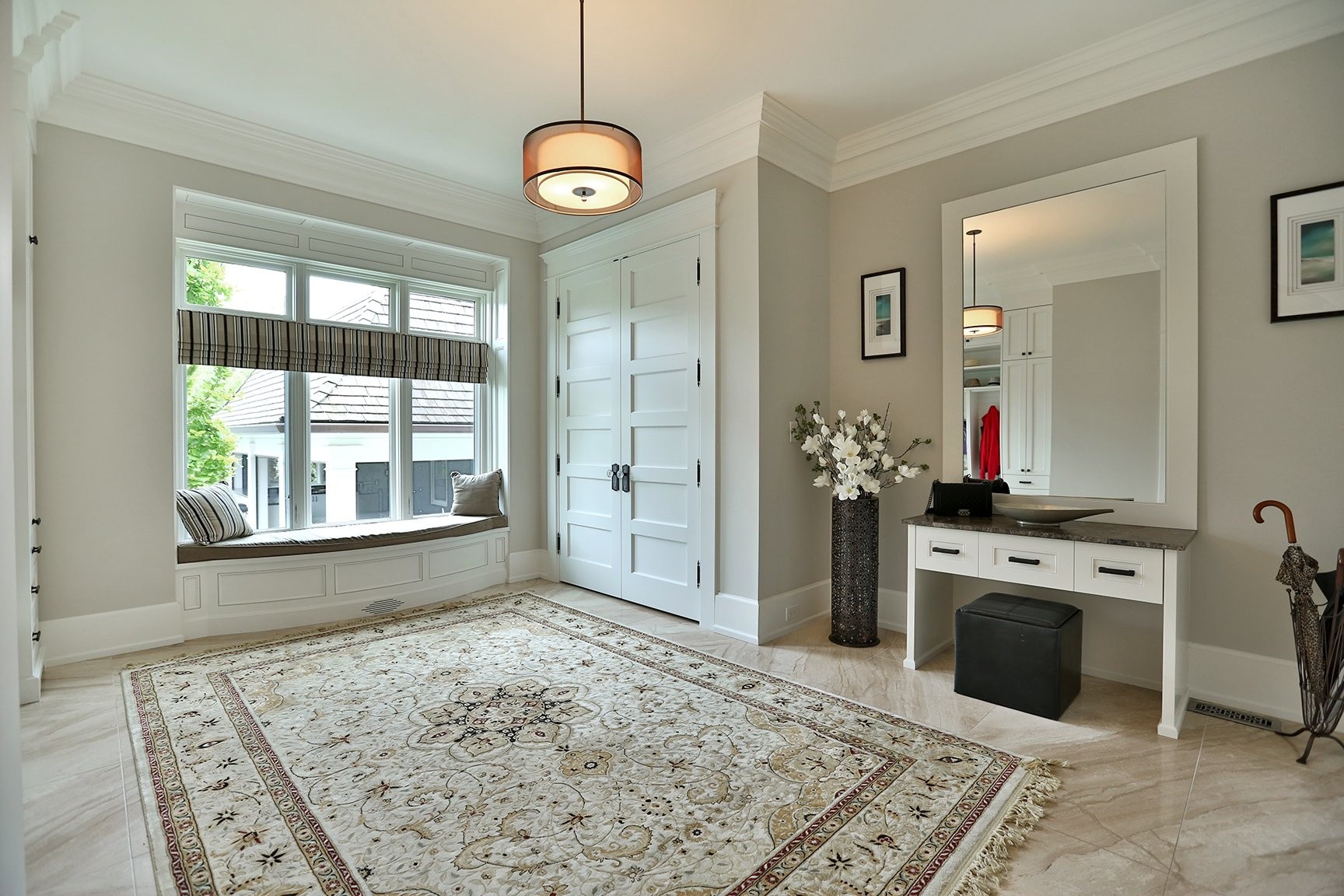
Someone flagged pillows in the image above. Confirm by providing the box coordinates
[176,480,254,546]
[449,469,503,515]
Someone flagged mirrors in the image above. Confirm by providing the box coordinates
[940,136,1200,549]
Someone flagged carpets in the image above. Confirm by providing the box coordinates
[120,591,1061,896]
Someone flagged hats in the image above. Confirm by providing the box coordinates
[964,360,981,367]
[986,377,1000,386]
[964,378,982,387]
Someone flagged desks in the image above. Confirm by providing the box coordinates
[900,512,1197,741]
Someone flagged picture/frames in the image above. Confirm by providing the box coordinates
[861,267,906,360]
[1268,181,1344,323]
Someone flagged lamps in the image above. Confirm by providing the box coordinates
[524,0,642,214]
[961,229,1004,338]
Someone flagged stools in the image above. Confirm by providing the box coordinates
[954,593,1082,721]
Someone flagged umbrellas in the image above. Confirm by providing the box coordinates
[1312,568,1344,726]
[1253,501,1326,728]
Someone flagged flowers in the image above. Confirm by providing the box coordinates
[791,401,933,499]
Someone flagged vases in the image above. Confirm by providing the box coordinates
[830,496,878,647]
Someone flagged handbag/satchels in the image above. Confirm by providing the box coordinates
[963,474,1010,494]
[925,479,993,524]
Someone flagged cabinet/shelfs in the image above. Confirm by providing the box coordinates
[964,304,1053,492]
